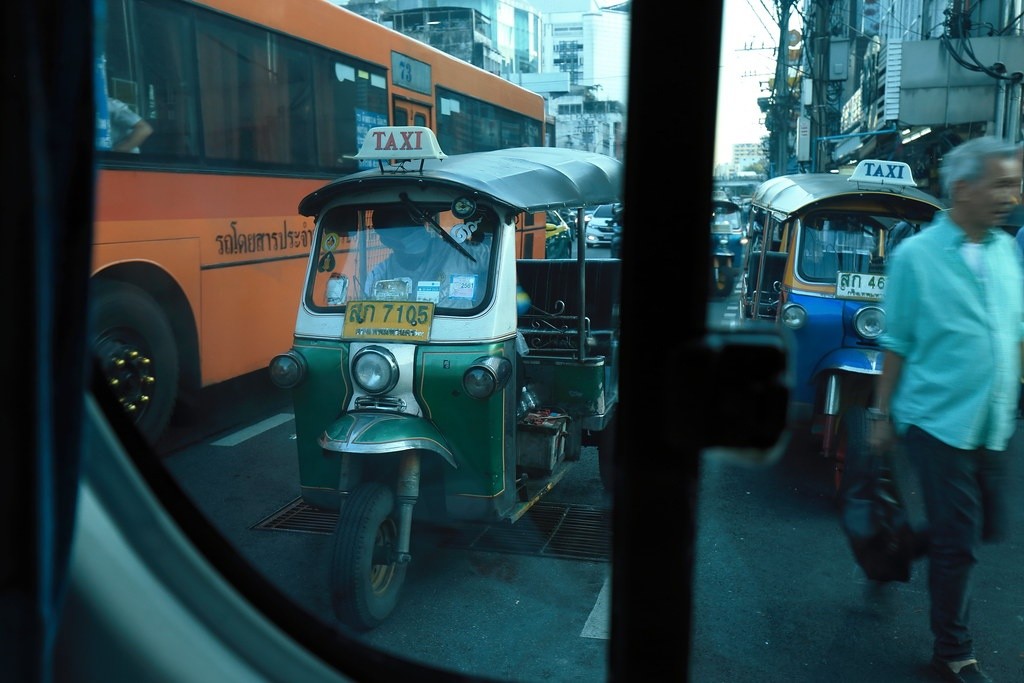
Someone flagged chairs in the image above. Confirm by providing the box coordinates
[822,251,871,278]
[749,251,789,302]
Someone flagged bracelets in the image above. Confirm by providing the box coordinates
[866,409,891,423]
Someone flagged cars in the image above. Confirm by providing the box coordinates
[545,209,570,260]
[586,203,622,248]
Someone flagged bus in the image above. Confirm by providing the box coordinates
[87,0,545,453]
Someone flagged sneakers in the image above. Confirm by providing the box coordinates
[930,653,994,682]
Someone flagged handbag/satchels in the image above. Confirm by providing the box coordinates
[841,448,920,585]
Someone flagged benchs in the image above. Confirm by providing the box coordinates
[517,259,619,360]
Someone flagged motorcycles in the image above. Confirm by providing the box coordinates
[267,126,621,627]
[710,190,744,295]
[606,219,621,259]
[742,160,945,509]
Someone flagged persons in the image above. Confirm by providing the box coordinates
[363,202,531,410]
[866,136,1024,683]
[607,202,623,232]
[106,94,152,153]
